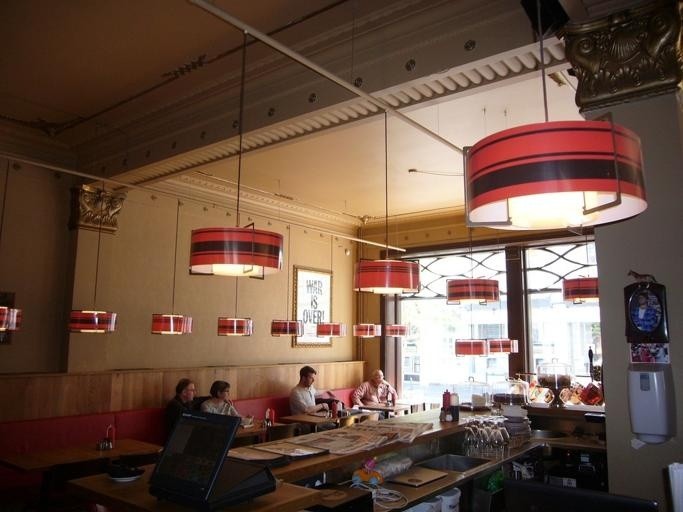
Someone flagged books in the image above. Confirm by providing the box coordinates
[250,417,432,461]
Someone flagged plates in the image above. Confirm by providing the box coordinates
[106,468,143,483]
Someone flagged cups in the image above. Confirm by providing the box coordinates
[500,462,513,485]
[470,394,485,407]
[343,410,347,417]
[96,441,112,450]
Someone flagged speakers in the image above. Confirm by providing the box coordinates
[520,0,570,34]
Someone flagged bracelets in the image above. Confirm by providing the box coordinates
[320,402,324,409]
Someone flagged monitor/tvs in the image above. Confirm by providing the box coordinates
[147,407,240,502]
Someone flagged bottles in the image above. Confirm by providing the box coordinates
[331,399,342,417]
[386,392,391,406]
[439,389,459,422]
[265,407,274,426]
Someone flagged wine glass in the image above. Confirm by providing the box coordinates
[461,414,510,460]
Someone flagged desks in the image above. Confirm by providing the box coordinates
[0,398,410,512]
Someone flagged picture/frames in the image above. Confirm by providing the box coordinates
[292,264,332,346]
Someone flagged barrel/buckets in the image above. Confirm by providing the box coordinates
[404,487,461,512]
[560,448,577,473]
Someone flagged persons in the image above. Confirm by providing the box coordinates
[162,377,233,443]
[200,380,253,420]
[288,365,337,433]
[631,291,658,330]
[351,369,397,419]
[630,343,665,363]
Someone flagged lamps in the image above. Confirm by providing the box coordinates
[488,306,510,352]
[562,226,600,302]
[353,243,376,337]
[464,0,648,231]
[69,180,118,333]
[270,225,302,338]
[0,159,22,331]
[446,228,500,304]
[456,228,487,354]
[187,32,282,279]
[151,198,192,337]
[354,115,419,294]
[386,295,410,338]
[217,214,255,338]
[315,237,346,337]
